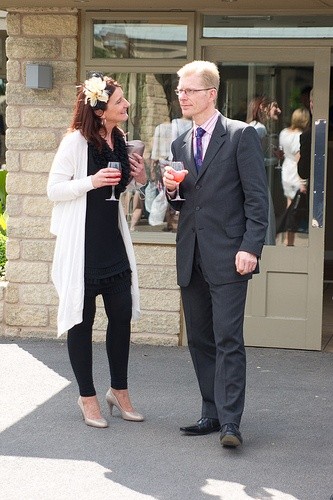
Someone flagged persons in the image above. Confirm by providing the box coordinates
[294,88,313,180]
[163,60,270,446]
[125,114,193,232]
[45,74,146,428]
[243,97,285,246]
[279,107,311,244]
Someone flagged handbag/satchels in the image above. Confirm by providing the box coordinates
[125,139,146,165]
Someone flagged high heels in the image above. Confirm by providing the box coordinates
[105,388,144,422]
[78,396,108,427]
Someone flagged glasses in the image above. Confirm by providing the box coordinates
[174,88,216,95]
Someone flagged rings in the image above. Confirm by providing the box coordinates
[134,167,136,170]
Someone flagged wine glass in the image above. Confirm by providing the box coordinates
[169,162,185,203]
[105,161,121,201]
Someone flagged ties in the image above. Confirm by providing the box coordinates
[193,128,208,170]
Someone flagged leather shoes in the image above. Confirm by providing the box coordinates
[181,417,220,436]
[218,423,243,447]
